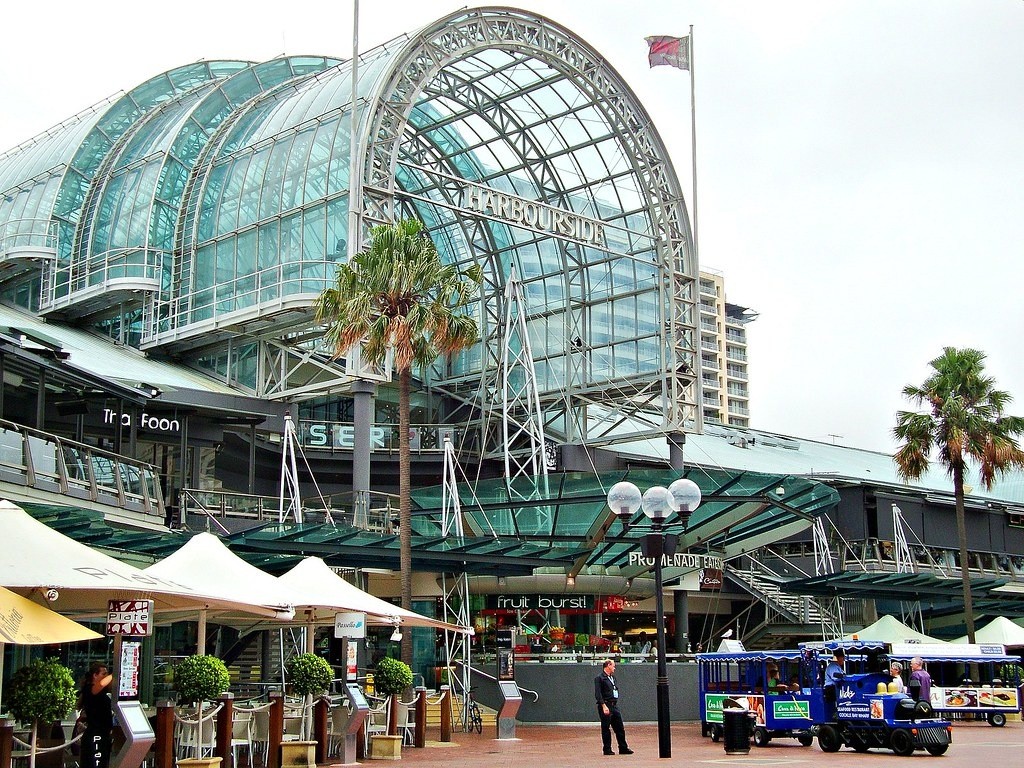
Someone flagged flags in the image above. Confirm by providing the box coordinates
[645,35,690,71]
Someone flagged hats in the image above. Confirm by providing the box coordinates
[833,649,847,657]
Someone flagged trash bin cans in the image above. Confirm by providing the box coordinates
[723,710,752,755]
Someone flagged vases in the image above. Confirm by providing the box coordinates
[550,632,563,639]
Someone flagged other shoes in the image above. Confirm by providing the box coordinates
[604,751,615,755]
[619,748,634,754]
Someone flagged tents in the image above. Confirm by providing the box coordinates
[952,616,1024,689]
[831,614,950,644]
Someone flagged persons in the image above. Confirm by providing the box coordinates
[594,660,634,754]
[891,662,905,693]
[907,657,931,750]
[349,648,356,673]
[636,632,657,663]
[767,659,824,694]
[70,663,113,768]
[695,643,702,653]
[480,629,497,653]
[824,651,845,700]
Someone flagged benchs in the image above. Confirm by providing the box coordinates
[709,681,788,696]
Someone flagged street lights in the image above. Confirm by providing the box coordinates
[604,478,704,759]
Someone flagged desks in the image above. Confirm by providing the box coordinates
[214,720,254,768]
[284,716,310,741]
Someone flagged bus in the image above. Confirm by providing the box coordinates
[819,653,1021,728]
[692,638,954,756]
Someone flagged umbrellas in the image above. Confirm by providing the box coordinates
[0,499,403,757]
[187,556,473,741]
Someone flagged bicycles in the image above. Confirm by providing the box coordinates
[457,686,484,734]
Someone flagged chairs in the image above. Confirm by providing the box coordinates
[806,660,836,703]
[143,703,416,768]
[64,746,80,768]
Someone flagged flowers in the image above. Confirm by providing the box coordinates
[549,626,565,632]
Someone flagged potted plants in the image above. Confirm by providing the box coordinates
[174,654,231,768]
[371,658,414,760]
[280,653,334,768]
